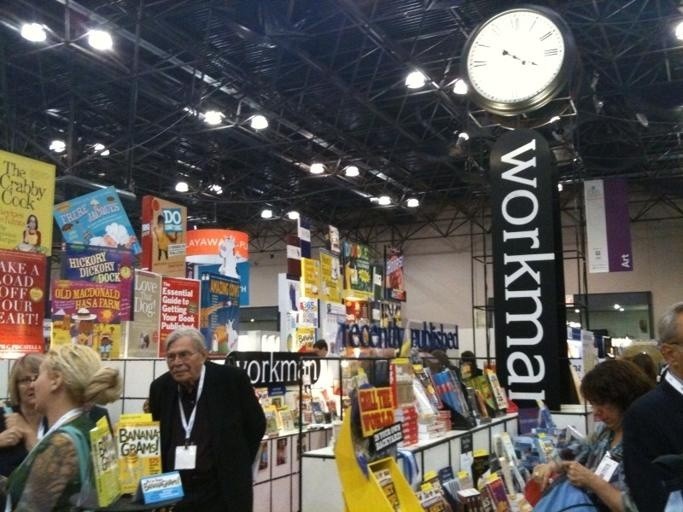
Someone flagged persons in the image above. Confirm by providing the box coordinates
[149,328,266,512]
[0,344,123,512]
[0,352,46,479]
[23,215,41,246]
[531,302,683,512]
[154,215,177,261]
[313,339,339,358]
[430,350,450,365]
[460,350,483,375]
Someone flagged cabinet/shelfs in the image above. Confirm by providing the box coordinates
[214,348,596,512]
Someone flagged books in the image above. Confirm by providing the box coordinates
[88,413,161,508]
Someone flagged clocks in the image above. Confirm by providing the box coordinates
[456,0,582,135]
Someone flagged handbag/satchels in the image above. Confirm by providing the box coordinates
[531,475,597,512]
